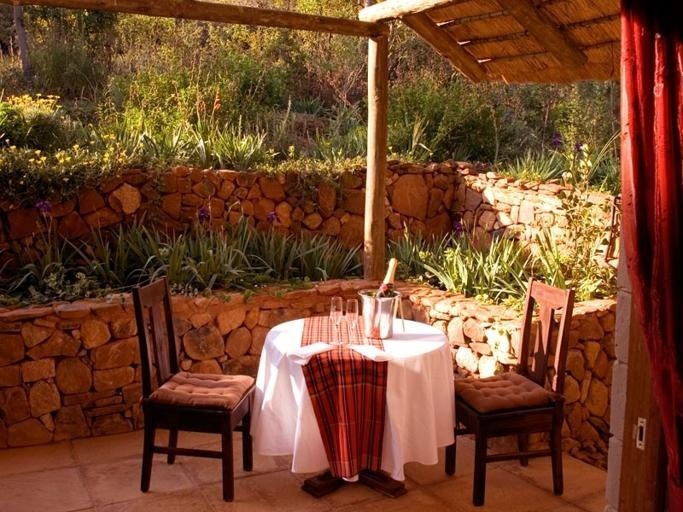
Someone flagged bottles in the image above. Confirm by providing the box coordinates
[376,257,398,297]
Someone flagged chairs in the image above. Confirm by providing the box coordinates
[448,275,574,507]
[128,276,255,504]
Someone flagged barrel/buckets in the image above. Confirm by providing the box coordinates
[359,289,405,340]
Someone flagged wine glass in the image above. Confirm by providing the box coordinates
[329,296,343,346]
[345,299,358,349]
